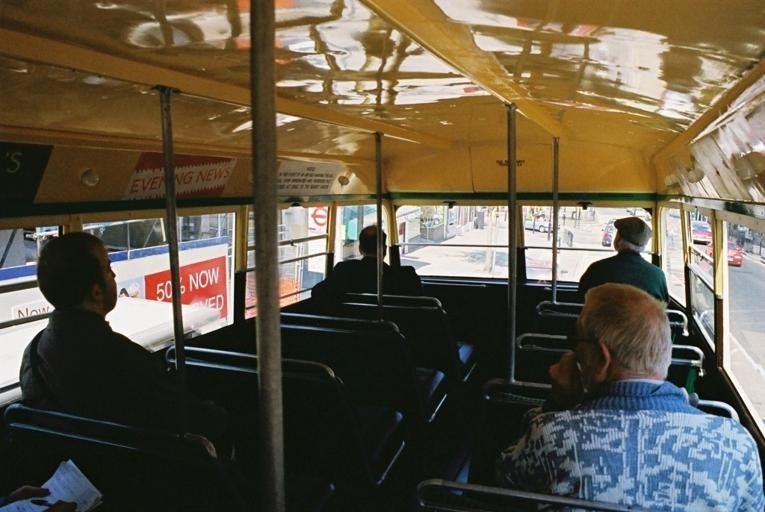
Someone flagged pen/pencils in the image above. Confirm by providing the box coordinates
[31,500,54,506]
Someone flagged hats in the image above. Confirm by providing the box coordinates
[614,217,651,246]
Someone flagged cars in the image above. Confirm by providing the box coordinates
[690,221,715,245]
[706,240,745,267]
[526,212,558,233]
[602,218,617,247]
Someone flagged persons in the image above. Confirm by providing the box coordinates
[491,279,765,512]
[0,484,82,511]
[18,230,234,467]
[574,216,672,314]
[310,220,425,301]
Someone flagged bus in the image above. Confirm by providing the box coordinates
[1,209,307,388]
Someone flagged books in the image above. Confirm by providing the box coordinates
[0,457,105,511]
